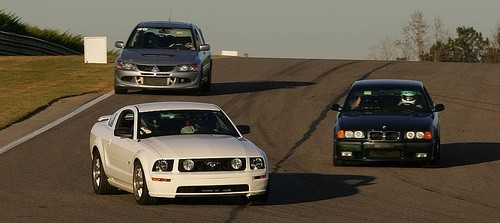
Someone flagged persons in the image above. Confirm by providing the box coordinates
[397,90,423,108]
[140,113,161,135]
[181,119,195,134]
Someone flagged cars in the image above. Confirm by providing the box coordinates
[88,101,271,205]
[114,20,213,96]
[329,79,445,168]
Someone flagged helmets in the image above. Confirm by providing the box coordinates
[401,90,416,105]
